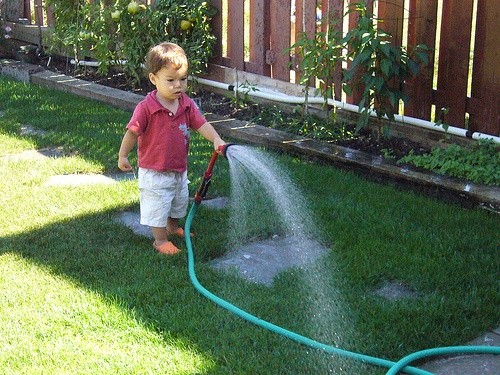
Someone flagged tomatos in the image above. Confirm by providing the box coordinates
[180,15,196,30]
[128,2,139,15]
[111,10,120,19]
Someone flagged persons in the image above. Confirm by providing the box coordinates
[117,41,229,256]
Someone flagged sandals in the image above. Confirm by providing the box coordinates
[153,241,181,255]
[166,227,194,238]
